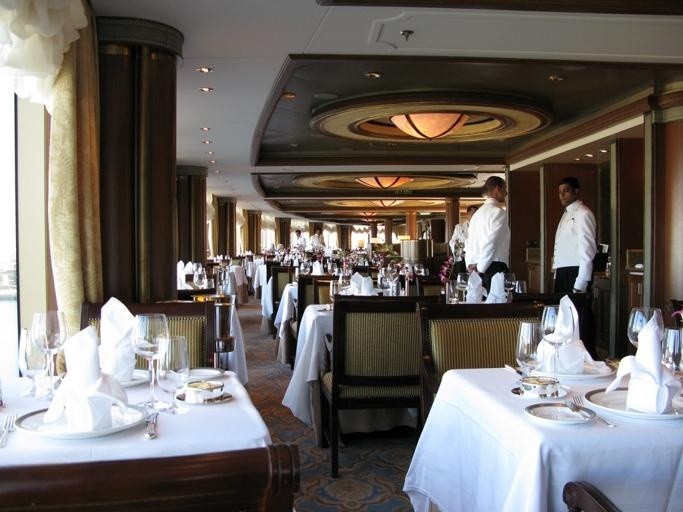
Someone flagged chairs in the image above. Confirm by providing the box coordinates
[562,481,621,511]
[671,298,682,325]
[319,294,447,478]
[81,303,216,368]
[416,301,546,424]
[402,365,682,511]
[0,444,301,511]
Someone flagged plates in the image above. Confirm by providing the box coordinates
[174,366,232,405]
[507,360,682,426]
[12,368,150,439]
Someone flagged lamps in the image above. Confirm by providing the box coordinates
[389,112,471,141]
[353,175,416,189]
[368,199,405,207]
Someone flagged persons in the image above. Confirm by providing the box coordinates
[449,206,478,274]
[551,177,598,349]
[293,229,306,253]
[463,176,512,301]
[310,228,325,253]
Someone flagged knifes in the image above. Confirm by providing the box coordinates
[144,412,158,440]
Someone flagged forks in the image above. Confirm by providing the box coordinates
[0,414,17,446]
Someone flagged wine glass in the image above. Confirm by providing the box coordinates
[192,263,206,289]
[514,304,575,379]
[295,262,310,282]
[132,313,190,417]
[327,263,351,309]
[627,306,682,374]
[376,261,424,297]
[445,273,527,304]
[18,311,68,398]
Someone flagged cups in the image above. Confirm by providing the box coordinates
[205,279,214,289]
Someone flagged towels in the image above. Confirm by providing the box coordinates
[99,296,137,380]
[536,295,596,375]
[604,310,680,414]
[46,325,111,432]
